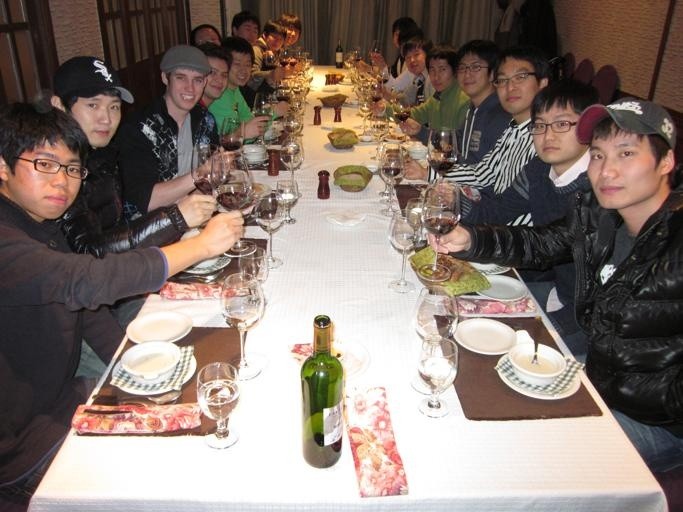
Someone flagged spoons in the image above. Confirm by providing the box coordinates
[530,316,542,368]
[90,391,180,405]
[170,273,218,285]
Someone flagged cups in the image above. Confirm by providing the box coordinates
[191,136,228,194]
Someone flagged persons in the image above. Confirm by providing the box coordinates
[369,16,472,132]
[403,39,554,227]
[437,78,601,357]
[0,102,244,512]
[400,39,513,218]
[190,11,302,142]
[113,45,235,215]
[428,96,683,475]
[50,55,218,379]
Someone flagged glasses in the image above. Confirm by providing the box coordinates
[528,120,576,135]
[492,73,535,87]
[456,63,489,72]
[16,156,88,179]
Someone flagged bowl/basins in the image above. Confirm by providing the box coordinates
[118,340,182,382]
[509,340,566,386]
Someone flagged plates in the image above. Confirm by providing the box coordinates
[108,349,196,396]
[454,294,538,318]
[467,263,511,274]
[181,253,230,274]
[497,353,582,401]
[122,310,192,346]
[477,274,527,302]
[452,317,517,355]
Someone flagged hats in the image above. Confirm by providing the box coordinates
[53,57,134,104]
[577,97,676,148]
[160,45,209,75]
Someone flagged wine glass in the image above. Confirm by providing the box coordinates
[237,248,268,287]
[251,42,418,204]
[253,193,284,274]
[380,152,401,219]
[275,182,298,225]
[195,362,241,449]
[209,151,251,238]
[419,184,461,283]
[218,273,266,379]
[417,334,459,419]
[387,209,423,296]
[411,284,459,365]
[428,126,458,209]
[407,199,426,236]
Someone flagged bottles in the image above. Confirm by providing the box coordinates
[299,314,341,469]
